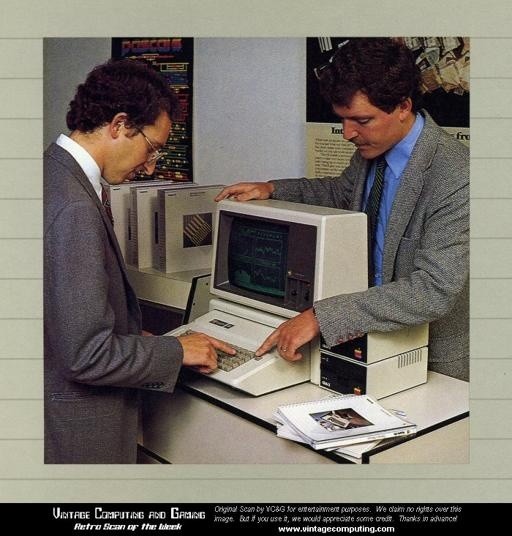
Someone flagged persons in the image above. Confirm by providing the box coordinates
[43,56,236,464]
[212,36,470,386]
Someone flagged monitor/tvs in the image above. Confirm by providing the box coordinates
[208,197,370,319]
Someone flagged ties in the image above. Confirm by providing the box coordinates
[365,158,386,243]
[99,182,114,225]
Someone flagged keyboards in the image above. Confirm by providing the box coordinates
[171,328,262,373]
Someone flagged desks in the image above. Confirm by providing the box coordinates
[114,261,470,469]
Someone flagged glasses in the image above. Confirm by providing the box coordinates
[133,124,164,164]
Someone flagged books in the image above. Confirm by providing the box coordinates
[274,390,420,462]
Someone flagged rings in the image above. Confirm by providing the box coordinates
[281,344,288,353]
